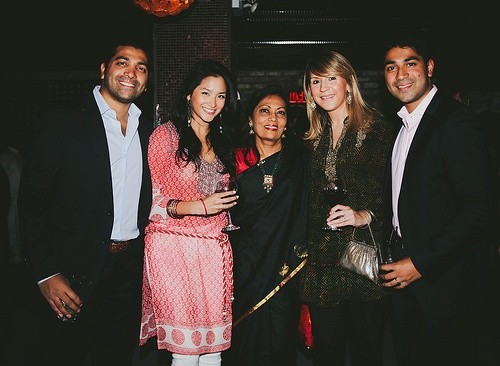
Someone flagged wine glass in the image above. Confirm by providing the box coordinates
[215,176,241,233]
[322,175,347,232]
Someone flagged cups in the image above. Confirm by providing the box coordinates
[385,258,397,281]
[57,274,92,322]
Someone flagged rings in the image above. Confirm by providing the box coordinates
[342,217,347,221]
[394,279,399,284]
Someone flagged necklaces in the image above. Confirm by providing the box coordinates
[258,146,283,193]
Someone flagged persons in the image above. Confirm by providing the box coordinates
[222,84,312,366]
[379,32,486,366]
[17,36,155,366]
[139,59,239,366]
[299,50,395,366]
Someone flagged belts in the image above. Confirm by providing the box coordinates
[108,239,140,256]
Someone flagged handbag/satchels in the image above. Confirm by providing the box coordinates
[339,210,383,285]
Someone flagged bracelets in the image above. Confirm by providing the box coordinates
[199,198,207,216]
